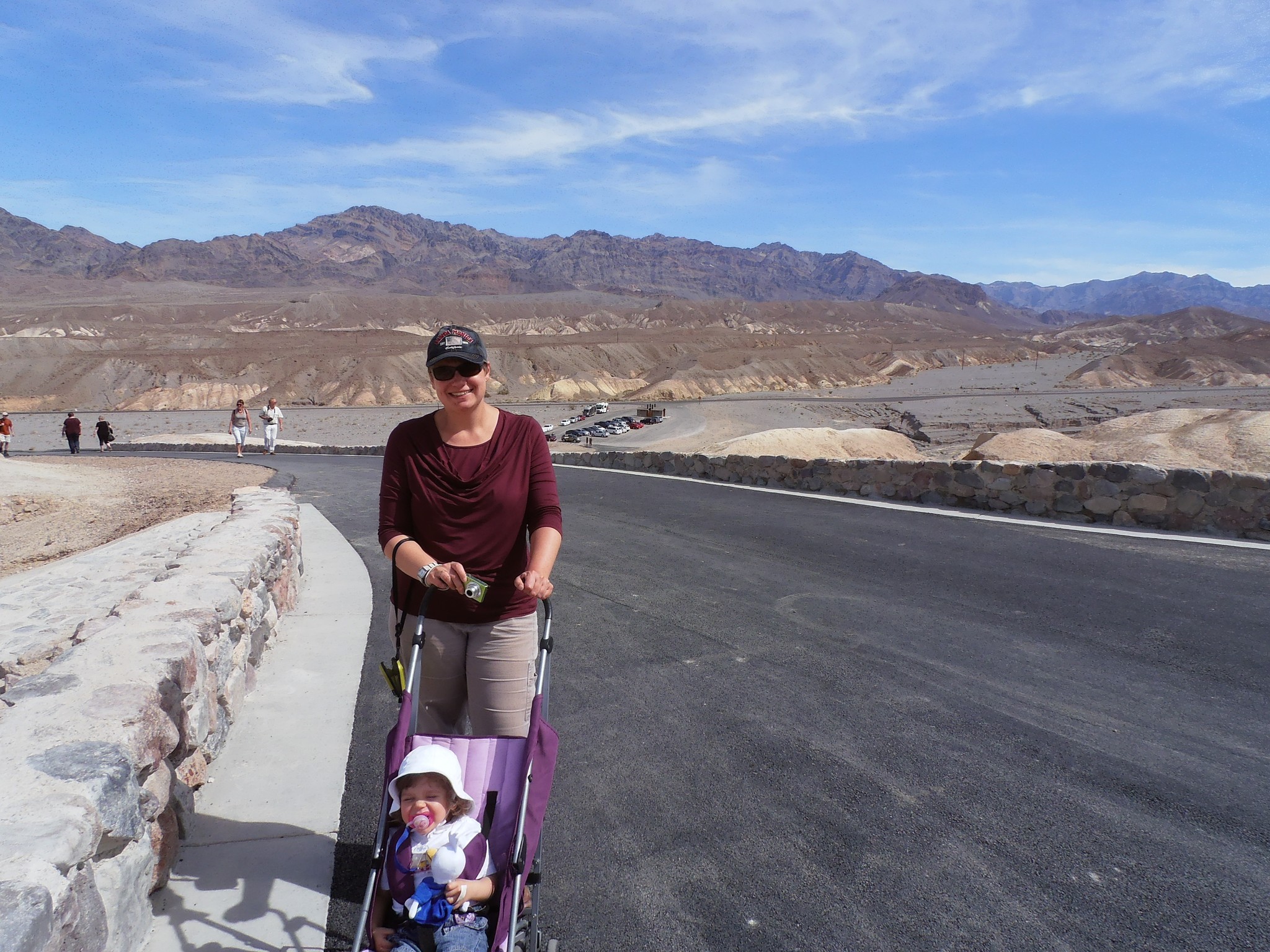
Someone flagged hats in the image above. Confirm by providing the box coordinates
[426,325,488,368]
[388,745,476,813]
[2,412,9,416]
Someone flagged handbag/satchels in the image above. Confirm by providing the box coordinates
[108,434,115,442]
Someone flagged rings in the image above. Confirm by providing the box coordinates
[439,570,446,577]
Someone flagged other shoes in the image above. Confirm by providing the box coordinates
[263,450,267,455]
[523,887,533,910]
[237,453,244,457]
[0,447,3,452]
[270,452,276,455]
[76,448,80,453]
[3,450,8,456]
[70,452,75,454]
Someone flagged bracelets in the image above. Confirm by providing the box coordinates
[281,425,283,427]
[93,434,96,435]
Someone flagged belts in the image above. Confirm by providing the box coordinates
[264,423,277,425]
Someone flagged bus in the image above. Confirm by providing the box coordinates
[582,402,609,417]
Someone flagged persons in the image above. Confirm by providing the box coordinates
[0,412,14,458]
[575,418,578,423]
[258,398,284,455]
[578,416,580,422]
[377,327,562,909]
[62,412,82,454]
[228,400,252,457]
[368,744,498,952]
[93,415,116,452]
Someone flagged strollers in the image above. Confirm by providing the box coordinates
[353,574,561,952]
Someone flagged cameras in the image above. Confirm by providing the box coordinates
[454,571,488,602]
[267,418,273,422]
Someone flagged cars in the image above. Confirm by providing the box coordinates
[543,424,554,432]
[639,416,664,425]
[562,416,644,443]
[561,415,585,426]
[545,434,557,442]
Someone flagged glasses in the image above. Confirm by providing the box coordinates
[430,361,484,382]
[237,404,243,406]
[272,401,278,404]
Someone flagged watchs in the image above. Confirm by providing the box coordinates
[417,562,439,588]
